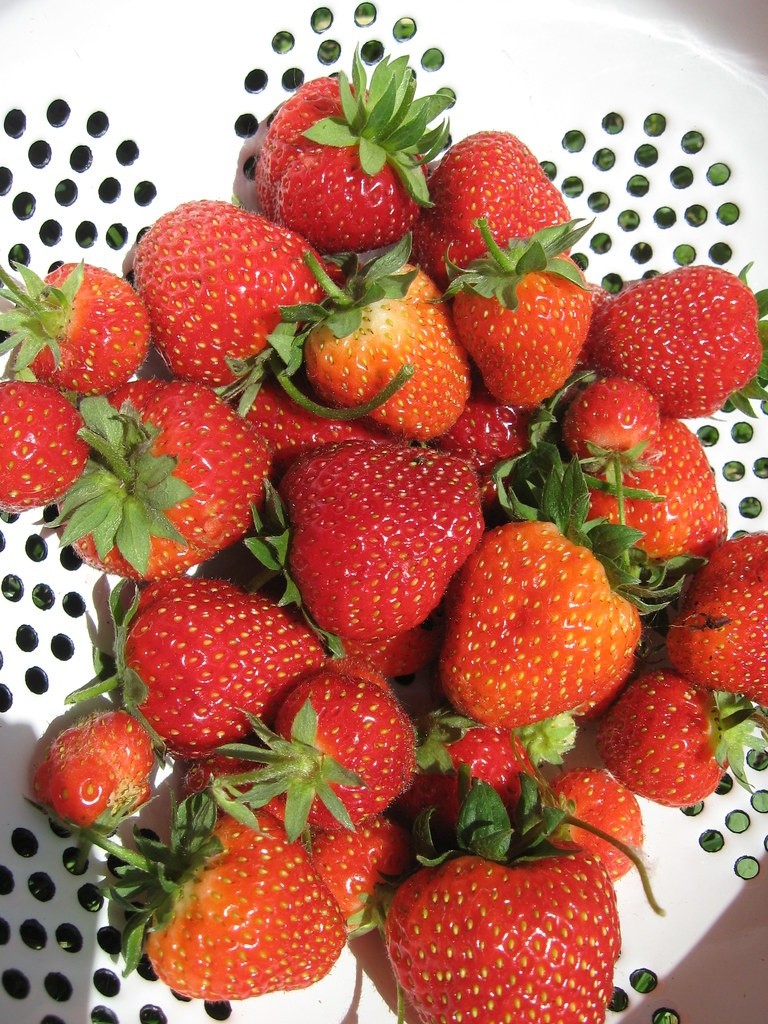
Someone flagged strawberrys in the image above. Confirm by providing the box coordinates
[0,76,768,1024]
